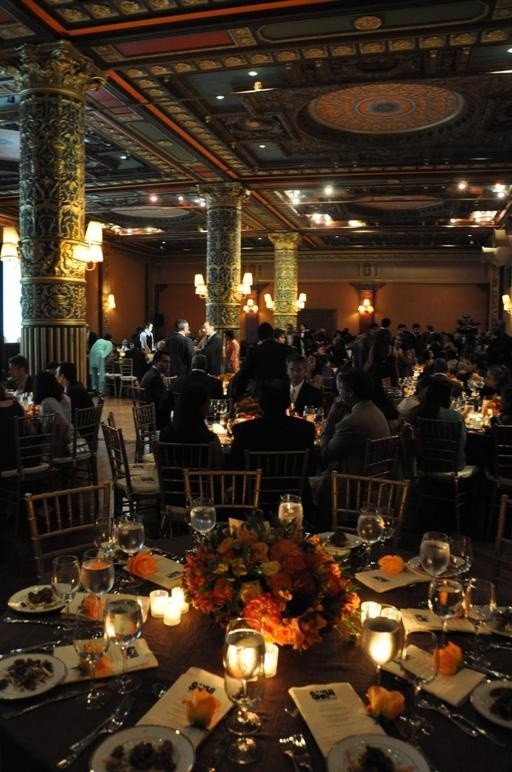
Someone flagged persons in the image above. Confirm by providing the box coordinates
[85,322,168,397]
[2,355,99,516]
[139,318,511,517]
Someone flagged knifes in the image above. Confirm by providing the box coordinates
[1,618,77,633]
[1,640,61,659]
[3,680,109,719]
[204,732,233,771]
[142,544,181,564]
[412,701,507,747]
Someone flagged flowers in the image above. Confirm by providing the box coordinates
[71,641,113,675]
[75,595,104,620]
[432,641,499,680]
[181,688,217,772]
[365,684,406,739]
[180,510,363,658]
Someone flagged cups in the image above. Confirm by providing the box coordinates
[260,643,280,678]
[359,600,383,623]
[303,404,325,425]
[147,584,190,627]
[6,388,34,406]
[279,493,304,531]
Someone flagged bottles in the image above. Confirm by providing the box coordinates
[398,376,414,397]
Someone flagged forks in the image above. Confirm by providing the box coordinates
[276,734,316,771]
[420,693,478,738]
[55,703,133,771]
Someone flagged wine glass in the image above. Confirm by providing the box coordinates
[207,398,231,424]
[189,495,218,542]
[221,615,267,737]
[53,514,151,708]
[360,603,405,686]
[417,530,497,662]
[400,629,442,700]
[377,507,399,552]
[356,504,386,559]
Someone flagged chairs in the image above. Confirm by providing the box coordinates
[2,392,104,515]
[496,495,511,546]
[24,481,112,584]
[105,360,117,397]
[99,397,509,534]
[330,469,409,549]
[118,358,141,399]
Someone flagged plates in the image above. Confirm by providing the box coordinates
[484,604,512,639]
[466,424,481,430]
[89,725,197,772]
[313,530,364,552]
[470,678,510,728]
[0,652,67,702]
[324,733,434,770]
[6,584,75,613]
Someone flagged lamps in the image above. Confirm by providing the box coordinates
[0,226,23,260]
[193,274,208,300]
[84,220,104,272]
[240,272,253,299]
